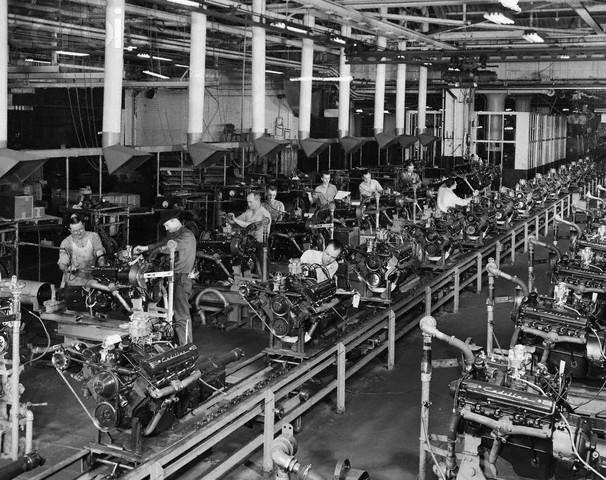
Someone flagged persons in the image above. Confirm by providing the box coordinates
[56,215,111,289]
[132,209,198,347]
[305,171,350,217]
[225,190,272,275]
[358,169,386,204]
[438,178,479,213]
[262,186,286,222]
[299,238,344,283]
[398,161,423,190]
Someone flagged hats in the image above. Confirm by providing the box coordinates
[157,207,181,226]
